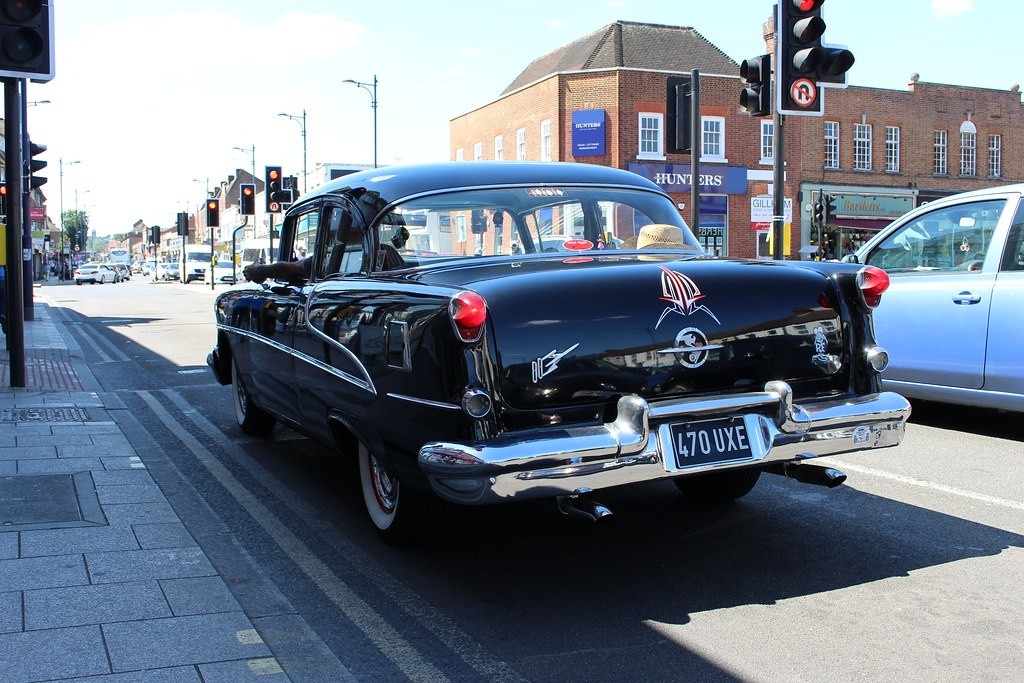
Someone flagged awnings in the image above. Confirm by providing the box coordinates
[42,249,54,257]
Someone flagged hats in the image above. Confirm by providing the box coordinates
[623,224,696,261]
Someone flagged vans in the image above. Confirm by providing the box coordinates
[178,243,212,284]
[238,238,295,282]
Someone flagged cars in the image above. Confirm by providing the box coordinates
[205,161,916,557]
[81,251,180,283]
[205,260,240,285]
[837,183,1024,414]
[73,263,118,285]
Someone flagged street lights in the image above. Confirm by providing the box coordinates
[341,74,378,168]
[277,109,307,194]
[192,178,209,239]
[75,188,92,268]
[60,158,81,282]
[233,144,257,240]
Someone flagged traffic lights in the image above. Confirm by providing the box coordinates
[822,194,836,224]
[264,165,294,213]
[777,0,856,116]
[206,198,220,228]
[739,54,772,117]
[813,202,824,222]
[240,183,256,215]
[0,0,56,81]
[0,182,16,182]
[29,141,49,190]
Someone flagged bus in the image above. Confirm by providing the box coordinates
[107,248,133,264]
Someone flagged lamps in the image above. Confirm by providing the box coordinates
[959,111,977,134]
